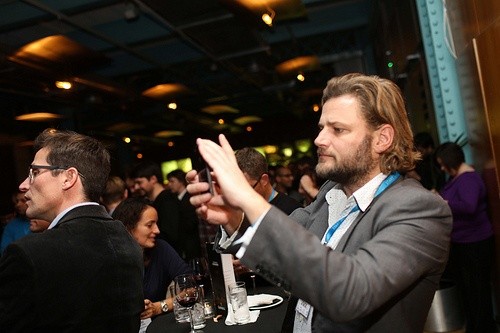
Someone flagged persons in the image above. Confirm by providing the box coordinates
[99,176,141,218]
[131,154,183,246]
[29,216,50,234]
[414,132,447,191]
[166,167,202,260]
[184,73,456,333]
[430,140,498,333]
[271,165,294,195]
[210,148,307,280]
[0,129,146,333]
[111,194,206,333]
[0,184,32,252]
[268,149,320,207]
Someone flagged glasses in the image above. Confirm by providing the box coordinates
[28,164,86,184]
[278,174,292,178]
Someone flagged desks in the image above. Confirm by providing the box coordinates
[146,280,289,333]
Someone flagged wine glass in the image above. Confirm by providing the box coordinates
[175,274,203,333]
[190,257,210,304]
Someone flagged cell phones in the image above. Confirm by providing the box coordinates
[198,168,214,196]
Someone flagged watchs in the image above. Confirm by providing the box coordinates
[160,300,169,313]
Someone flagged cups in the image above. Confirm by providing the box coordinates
[195,274,215,320]
[228,281,250,323]
[170,284,191,323]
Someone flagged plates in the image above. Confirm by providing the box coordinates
[246,293,284,309]
